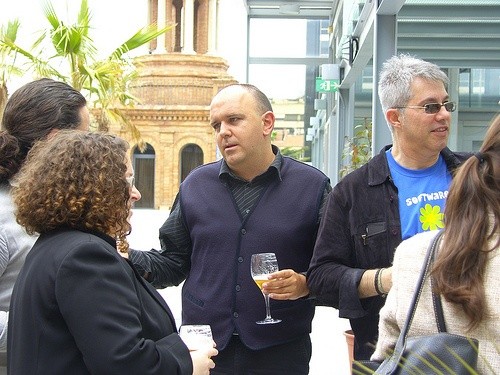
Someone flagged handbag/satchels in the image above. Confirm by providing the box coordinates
[372,228,479,375]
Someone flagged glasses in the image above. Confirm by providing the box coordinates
[125,177,135,190]
[390,101,456,114]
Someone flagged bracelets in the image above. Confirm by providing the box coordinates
[374,266,389,297]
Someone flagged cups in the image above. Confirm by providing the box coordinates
[180,324,214,360]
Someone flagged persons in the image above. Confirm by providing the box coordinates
[5,129,219,375]
[305,51,491,362]
[371,112,500,375]
[0,76,90,375]
[126,81,336,375]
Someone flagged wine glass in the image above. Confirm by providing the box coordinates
[251,253,282,324]
